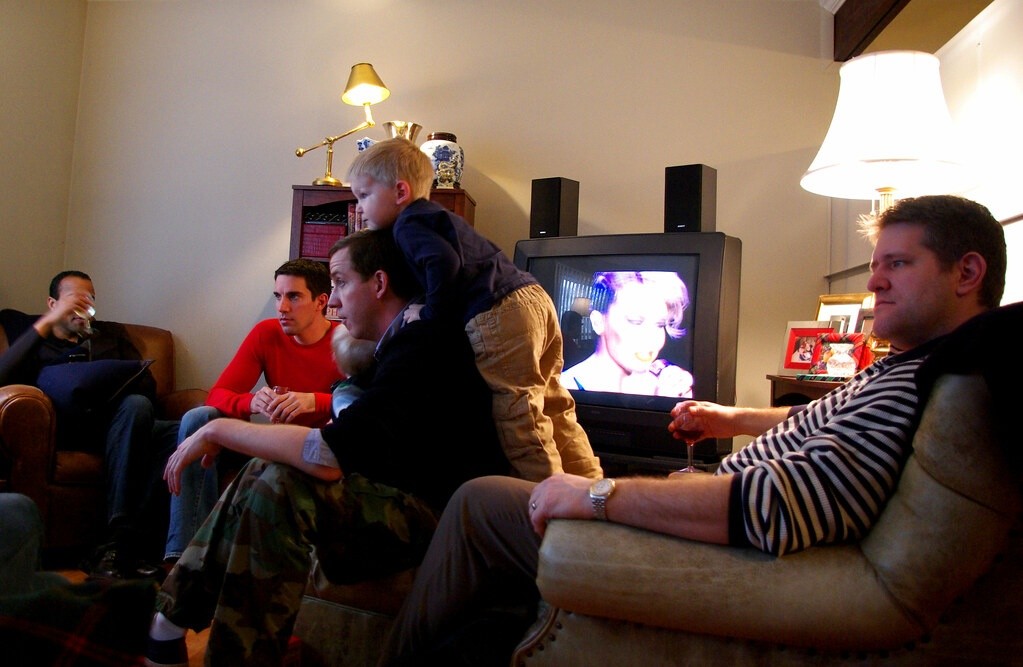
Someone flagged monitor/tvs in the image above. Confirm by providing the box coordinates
[512,231,743,463]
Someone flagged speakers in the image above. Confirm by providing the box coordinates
[530,176,580,239]
[664,164,717,232]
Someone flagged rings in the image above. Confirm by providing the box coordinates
[531,501,537,511]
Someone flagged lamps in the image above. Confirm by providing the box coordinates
[296,63,390,187]
[801,46,948,221]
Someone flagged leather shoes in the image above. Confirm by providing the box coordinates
[94,548,127,585]
[125,560,167,580]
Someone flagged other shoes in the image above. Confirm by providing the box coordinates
[119,611,190,667]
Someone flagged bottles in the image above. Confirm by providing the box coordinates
[827,343,855,376]
[272,385,288,425]
[419,131,465,184]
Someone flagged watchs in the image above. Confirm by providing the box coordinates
[590,477,617,523]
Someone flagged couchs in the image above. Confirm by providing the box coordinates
[512,300,1023,667]
[0,308,206,552]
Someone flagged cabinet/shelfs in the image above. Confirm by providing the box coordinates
[289,183,477,263]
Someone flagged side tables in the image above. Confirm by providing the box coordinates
[767,374,850,412]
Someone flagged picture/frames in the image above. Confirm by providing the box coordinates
[856,308,892,335]
[811,332,866,376]
[777,318,846,374]
[814,292,870,333]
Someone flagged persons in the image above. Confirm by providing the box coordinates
[793,336,815,361]
[0,272,158,586]
[97,233,512,667]
[557,273,694,399]
[163,259,346,573]
[346,139,605,483]
[376,196,1007,667]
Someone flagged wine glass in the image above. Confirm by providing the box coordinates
[73,289,100,337]
[675,402,704,473]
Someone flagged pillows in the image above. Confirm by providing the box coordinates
[37,358,156,419]
